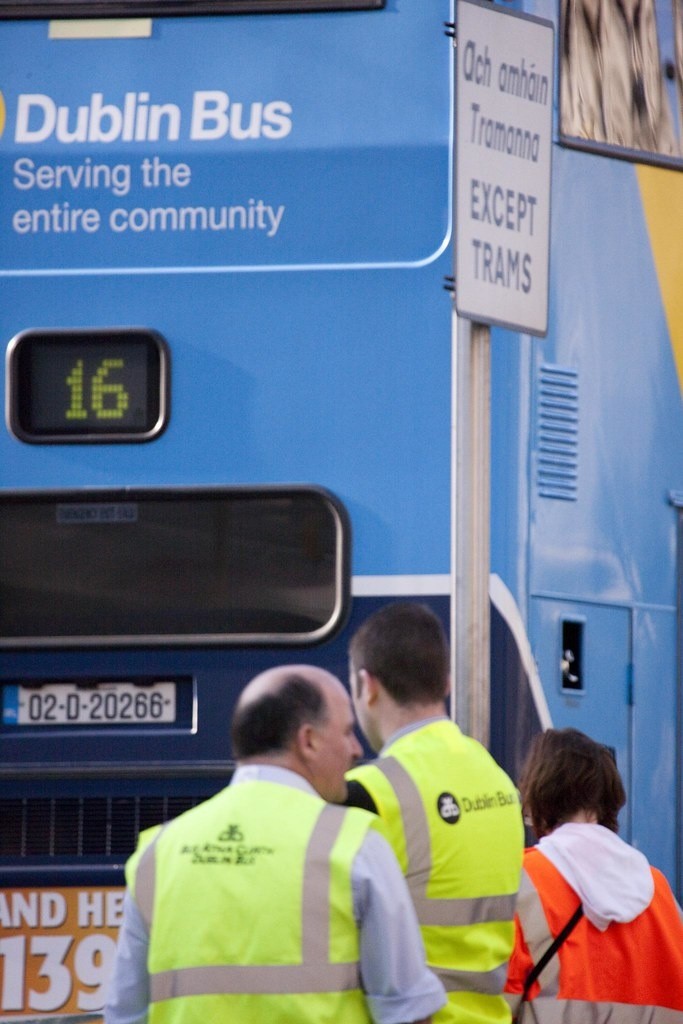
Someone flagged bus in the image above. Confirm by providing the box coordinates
[0,0,683,1024]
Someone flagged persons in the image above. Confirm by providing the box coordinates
[341,602,525,1024]
[504,729,683,1024]
[105,664,447,1023]
